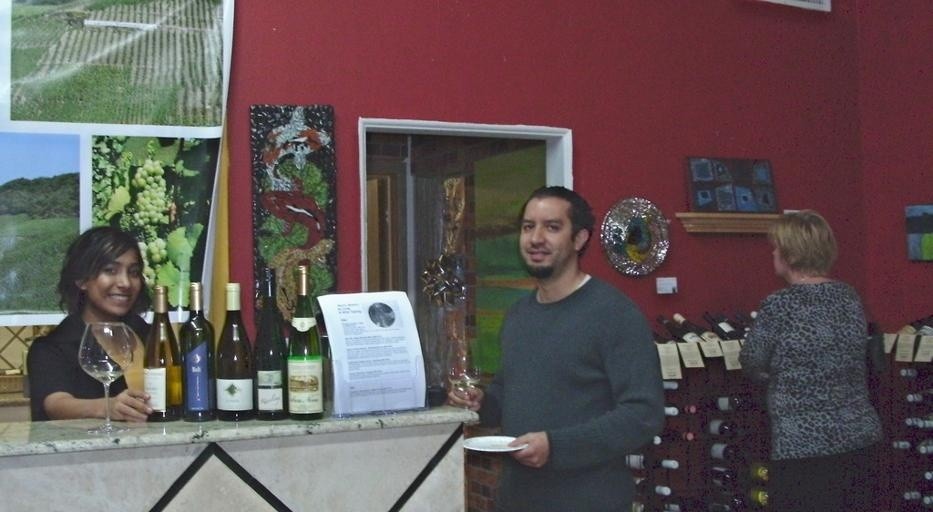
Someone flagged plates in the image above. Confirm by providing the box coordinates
[459,437,529,454]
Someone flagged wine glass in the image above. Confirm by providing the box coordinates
[447,346,482,440]
[79,320,134,434]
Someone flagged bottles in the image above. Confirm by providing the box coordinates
[142,263,327,425]
[649,309,763,343]
[892,370,932,512]
[626,390,771,512]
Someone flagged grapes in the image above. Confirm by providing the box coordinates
[131,157,172,287]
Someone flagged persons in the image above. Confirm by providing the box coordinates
[737,207,887,512]
[25,226,153,423]
[446,184,667,512]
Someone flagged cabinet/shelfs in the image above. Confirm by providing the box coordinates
[631,332,932,510]
